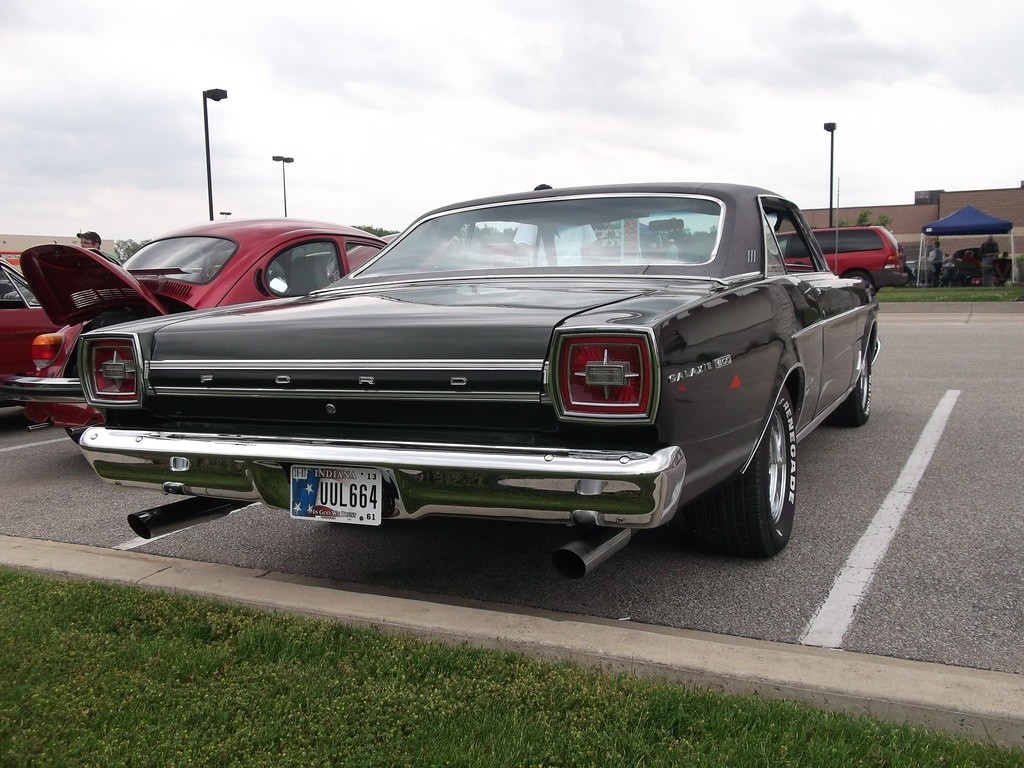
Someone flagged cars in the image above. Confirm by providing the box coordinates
[76,179,882,583]
[762,226,909,301]
[0,252,66,403]
[1,219,392,442]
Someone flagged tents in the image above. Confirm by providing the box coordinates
[917,205,1014,288]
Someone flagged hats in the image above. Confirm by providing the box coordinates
[76,231,101,245]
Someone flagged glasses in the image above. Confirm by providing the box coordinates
[80,241,95,246]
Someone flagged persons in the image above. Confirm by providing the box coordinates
[957,250,977,285]
[942,252,961,285]
[514,184,605,268]
[667,238,680,262]
[76,231,101,251]
[926,241,944,287]
[980,236,999,285]
[993,251,1011,285]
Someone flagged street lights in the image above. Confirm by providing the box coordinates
[823,121,839,226]
[273,154,295,220]
[202,88,228,222]
